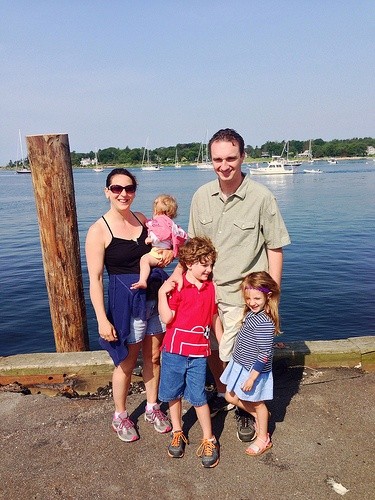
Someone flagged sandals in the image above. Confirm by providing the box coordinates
[254,412,272,431]
[245,433,273,456]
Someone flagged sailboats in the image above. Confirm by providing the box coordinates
[140,135,159,171]
[197,129,213,170]
[14,130,32,174]
[94,150,103,172]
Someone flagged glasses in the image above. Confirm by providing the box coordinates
[107,183,136,194]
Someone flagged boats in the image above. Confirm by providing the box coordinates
[268,141,301,166]
[304,169,323,173]
[249,161,294,175]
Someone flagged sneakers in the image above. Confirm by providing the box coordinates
[168,429,188,458]
[208,394,235,418]
[196,435,220,468]
[234,408,257,443]
[112,411,139,441]
[145,403,172,433]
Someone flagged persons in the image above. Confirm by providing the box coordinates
[168,128,291,443]
[220,271,283,456]
[85,168,172,441]
[130,195,189,290]
[158,238,224,468]
